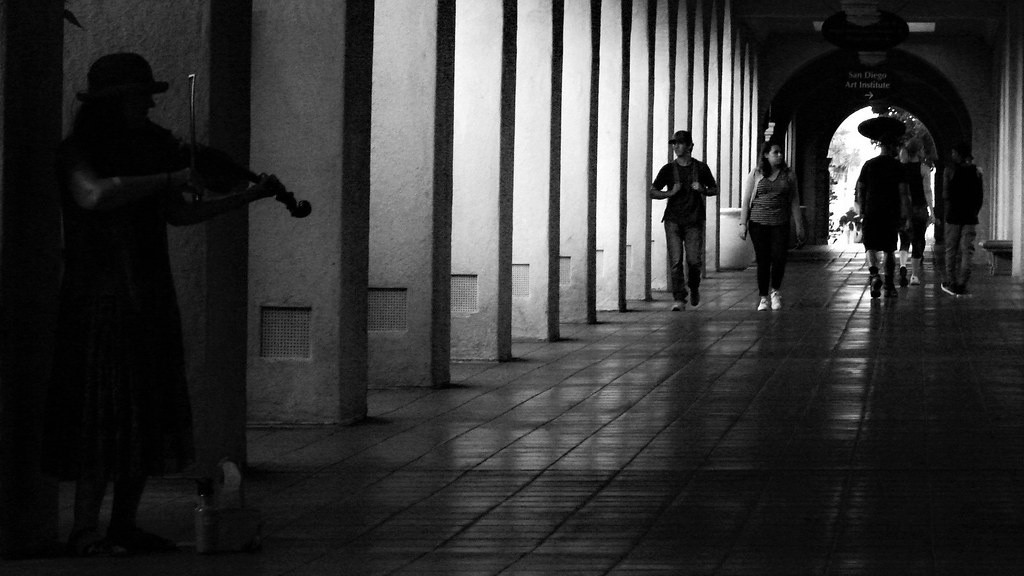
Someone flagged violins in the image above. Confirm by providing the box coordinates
[122,117,312,219]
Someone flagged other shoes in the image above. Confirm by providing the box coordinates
[770,291,782,309]
[883,275,899,298]
[900,265,908,287]
[671,301,685,311]
[757,297,768,311]
[941,280,968,297]
[910,275,920,284]
[870,276,883,298]
[690,289,700,306]
[106,525,177,552]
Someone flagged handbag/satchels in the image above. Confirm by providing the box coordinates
[739,216,750,241]
[854,231,864,243]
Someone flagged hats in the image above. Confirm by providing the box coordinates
[76,52,169,100]
[668,130,692,144]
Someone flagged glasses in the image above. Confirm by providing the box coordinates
[672,141,683,146]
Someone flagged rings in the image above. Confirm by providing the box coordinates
[694,186,695,187]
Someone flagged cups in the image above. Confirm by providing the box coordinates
[195,506,221,553]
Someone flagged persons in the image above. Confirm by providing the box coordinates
[649,131,720,312]
[57,52,283,562]
[853,134,914,298]
[940,142,984,299]
[739,141,808,311]
[895,137,931,286]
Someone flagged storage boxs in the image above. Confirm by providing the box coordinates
[194,505,262,552]
